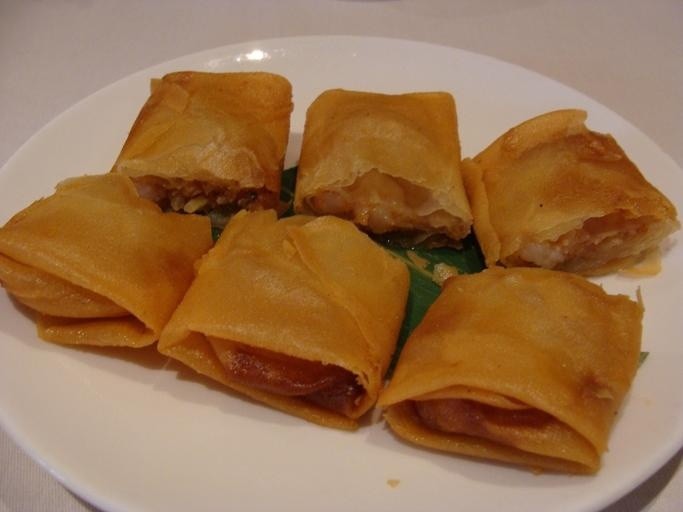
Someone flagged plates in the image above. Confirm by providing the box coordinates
[0,33,682,509]
